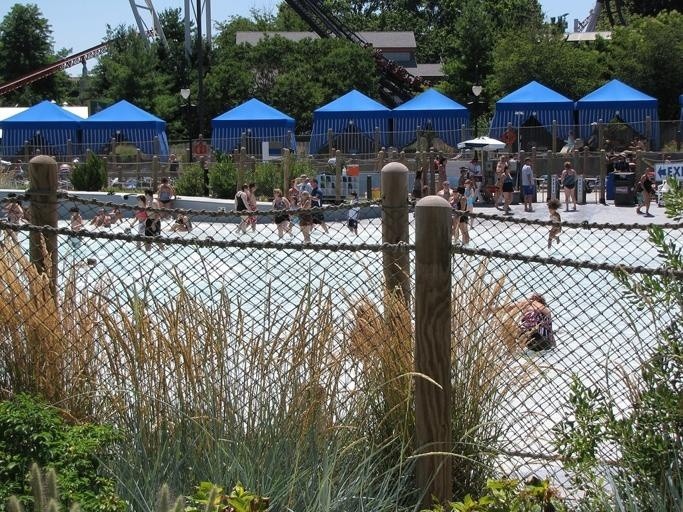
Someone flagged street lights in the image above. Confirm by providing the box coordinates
[181,81,193,162]
[515,111,525,161]
[467,80,488,138]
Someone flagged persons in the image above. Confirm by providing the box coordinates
[482,293,556,355]
[547,198,562,249]
[409,144,534,245]
[234,147,399,244]
[561,137,672,217]
[1,134,221,266]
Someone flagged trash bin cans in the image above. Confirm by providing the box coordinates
[614,172,636,207]
[607,172,614,200]
